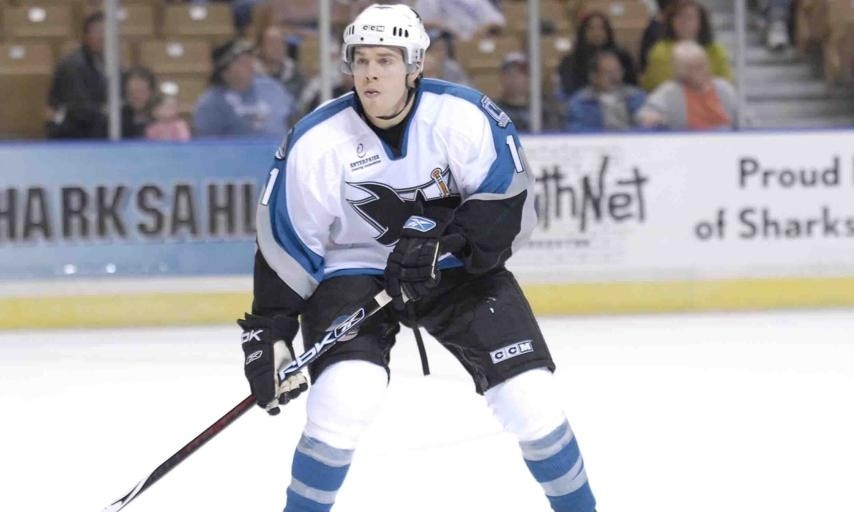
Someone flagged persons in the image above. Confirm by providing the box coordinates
[489,52,561,134]
[252,22,311,106]
[755,1,797,53]
[192,39,302,139]
[638,0,681,75]
[143,93,192,142]
[641,1,733,89]
[47,11,105,139]
[565,49,648,131]
[234,0,602,512]
[630,39,761,129]
[557,7,638,93]
[121,67,157,139]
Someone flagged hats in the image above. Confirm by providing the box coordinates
[202,38,256,87]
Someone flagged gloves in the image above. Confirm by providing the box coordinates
[378,198,460,317]
[237,311,308,417]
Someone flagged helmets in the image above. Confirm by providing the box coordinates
[339,3,433,66]
